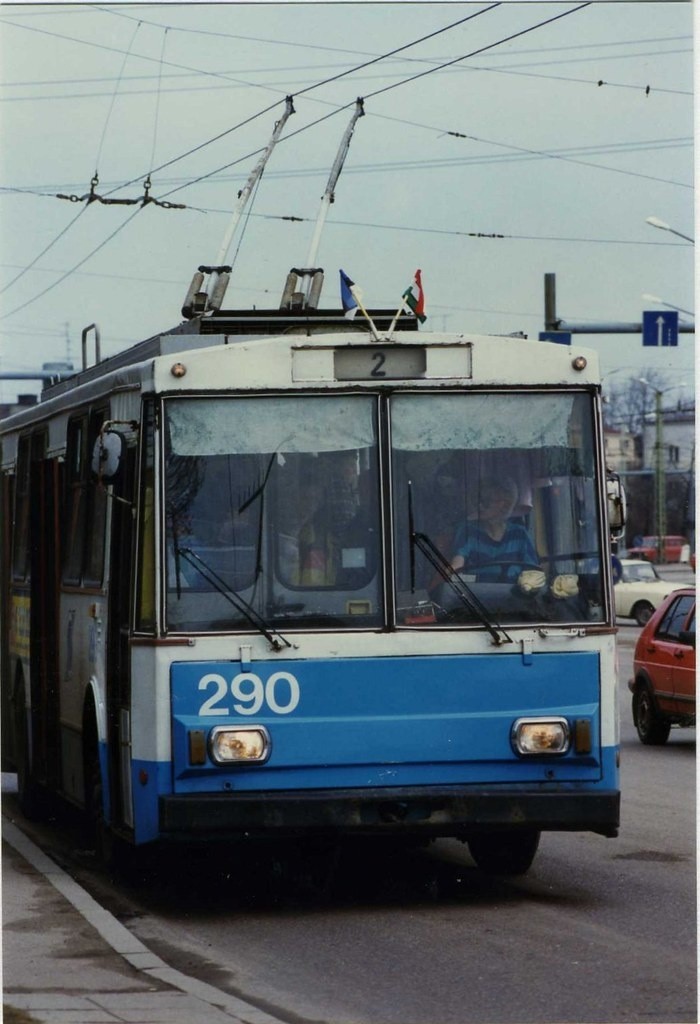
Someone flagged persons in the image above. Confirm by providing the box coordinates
[191,452,375,584]
[433,470,541,582]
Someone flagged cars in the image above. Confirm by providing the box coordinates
[614,560,696,627]
[628,536,687,563]
[632,589,696,745]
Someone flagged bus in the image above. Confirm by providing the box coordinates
[1,95,620,879]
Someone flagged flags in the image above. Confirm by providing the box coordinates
[400,269,427,324]
[339,270,362,318]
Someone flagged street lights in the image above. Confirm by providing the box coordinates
[638,378,689,561]
